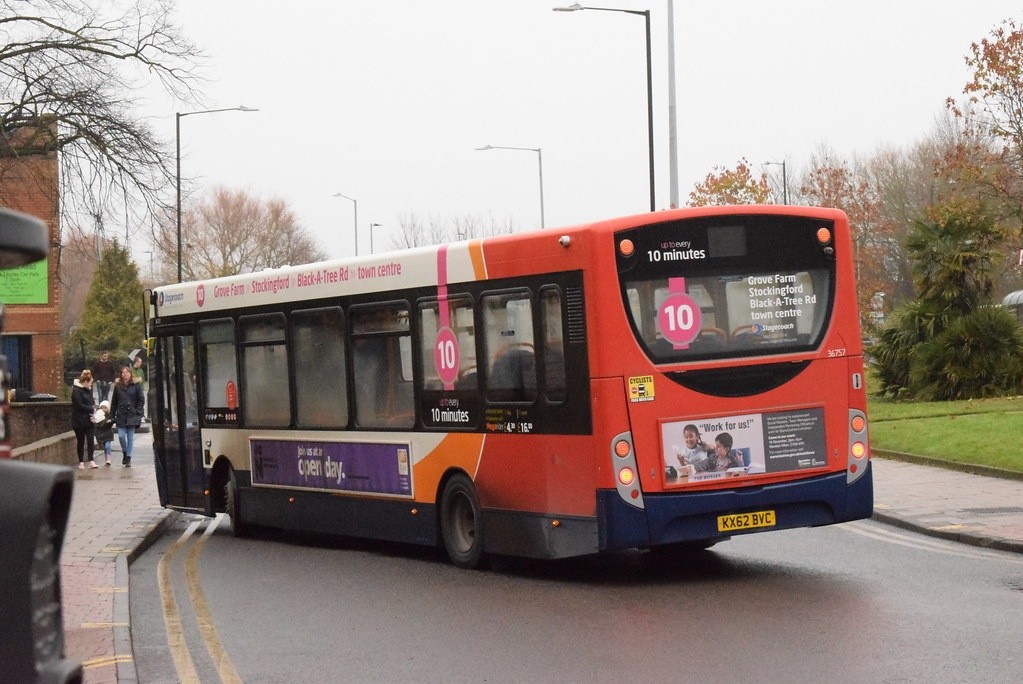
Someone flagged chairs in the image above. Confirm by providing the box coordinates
[427,324,774,396]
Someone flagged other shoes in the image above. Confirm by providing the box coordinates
[88,461,98,469]
[126,457,132,467]
[122,452,126,464]
[78,462,85,469]
[106,460,111,465]
[141,417,145,422]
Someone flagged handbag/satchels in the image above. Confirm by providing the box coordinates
[89,407,105,424]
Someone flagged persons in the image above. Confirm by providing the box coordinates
[109,366,145,467]
[127,357,151,423]
[679,432,745,474]
[676,424,715,466]
[169,366,195,418]
[89,350,115,404]
[71,369,99,469]
[91,400,117,465]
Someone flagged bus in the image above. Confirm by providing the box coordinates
[141,205,877,569]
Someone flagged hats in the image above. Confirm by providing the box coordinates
[99,400,110,410]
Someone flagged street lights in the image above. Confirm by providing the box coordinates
[763,159,787,205]
[175,105,260,284]
[370,223,382,254]
[552,2,656,211]
[332,193,358,256]
[474,144,545,229]
